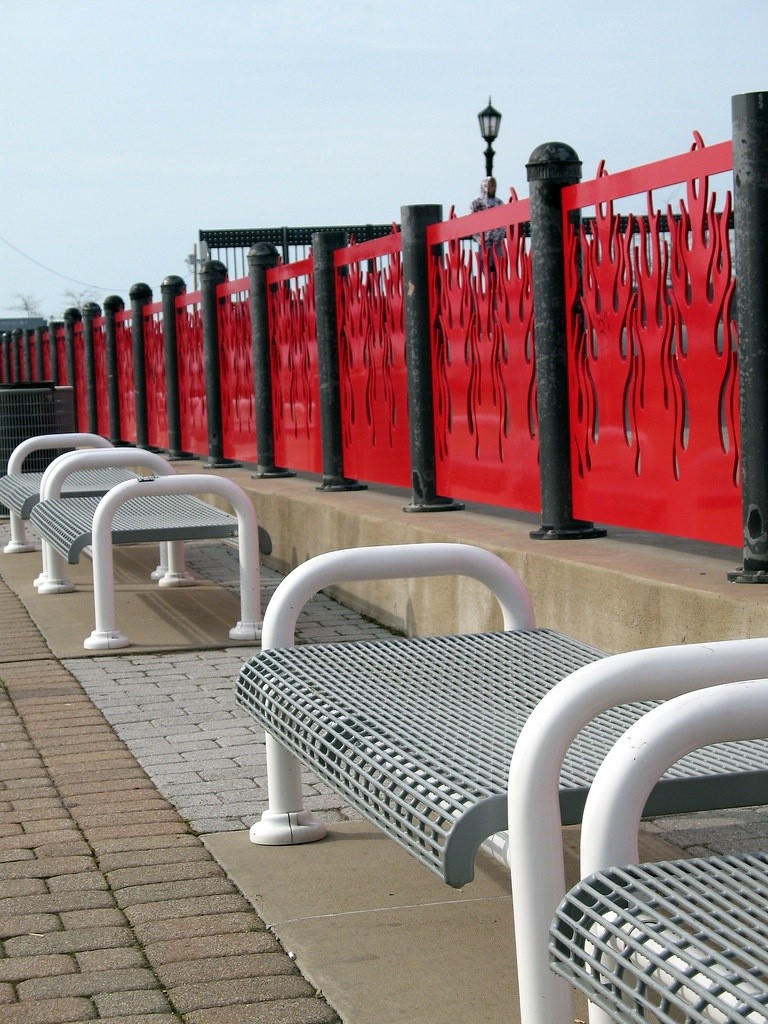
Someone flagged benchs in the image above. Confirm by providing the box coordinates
[0,432,169,581]
[28,451,273,650]
[548,677,768,1024]
[233,542,768,1024]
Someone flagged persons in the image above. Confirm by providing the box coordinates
[471,177,510,273]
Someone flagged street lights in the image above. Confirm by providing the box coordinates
[478,94,503,186]
